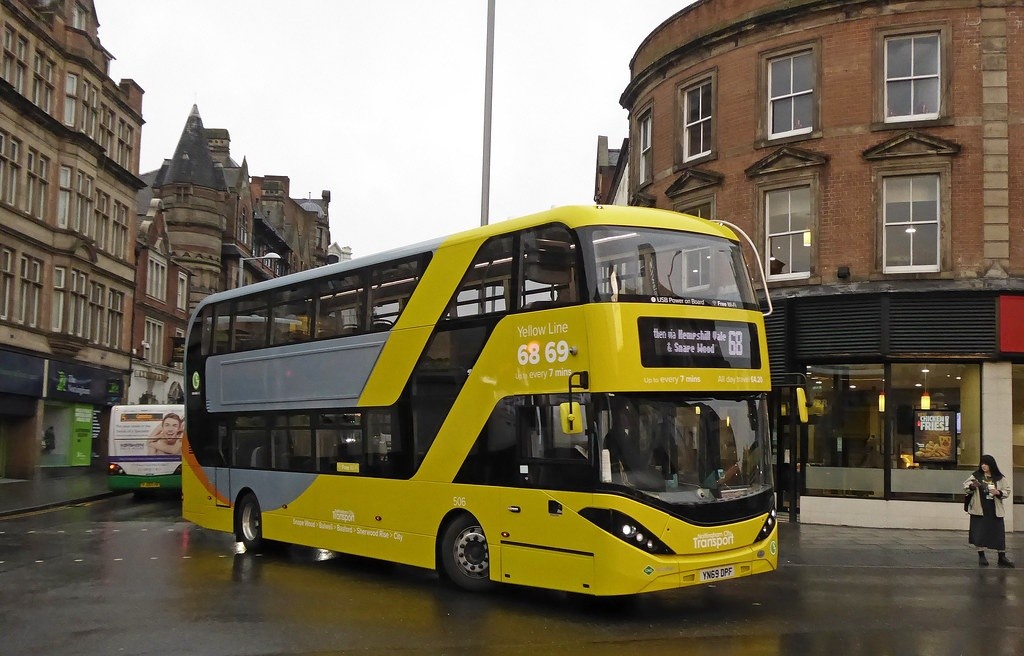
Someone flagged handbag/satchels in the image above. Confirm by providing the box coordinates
[964,494,973,512]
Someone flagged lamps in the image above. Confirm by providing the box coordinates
[804,213,811,247]
[837,267,850,279]
[920,364,930,409]
[879,365,885,412]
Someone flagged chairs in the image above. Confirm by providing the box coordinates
[276,320,393,346]
[235,445,404,477]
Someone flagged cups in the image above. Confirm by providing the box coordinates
[988,485,995,496]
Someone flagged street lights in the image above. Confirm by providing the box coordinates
[238,251,281,289]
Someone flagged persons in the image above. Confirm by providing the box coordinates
[145,413,185,456]
[962,455,1015,567]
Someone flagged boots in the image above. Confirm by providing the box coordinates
[998,552,1014,567]
[978,550,989,566]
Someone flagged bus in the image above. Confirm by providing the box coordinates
[181,209,816,599]
[105,404,186,495]
